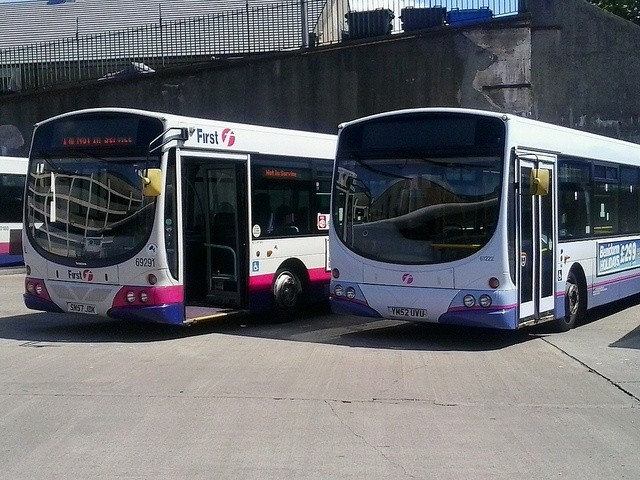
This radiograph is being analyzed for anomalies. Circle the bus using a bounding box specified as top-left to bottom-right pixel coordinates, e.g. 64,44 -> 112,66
22,107 -> 338,327
0,157 -> 29,267
329,107 -> 640,332
